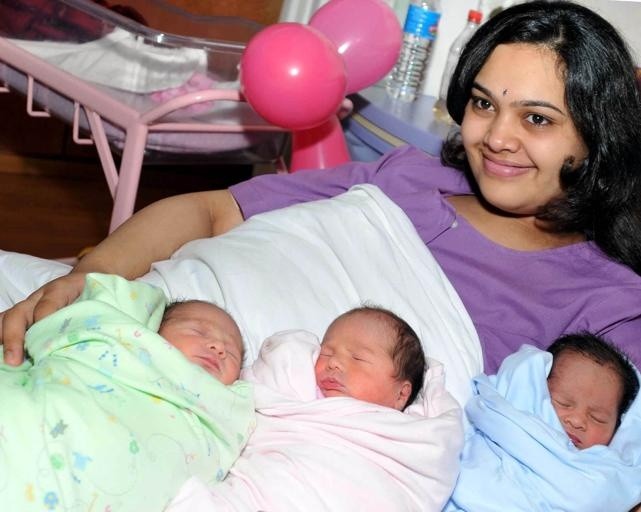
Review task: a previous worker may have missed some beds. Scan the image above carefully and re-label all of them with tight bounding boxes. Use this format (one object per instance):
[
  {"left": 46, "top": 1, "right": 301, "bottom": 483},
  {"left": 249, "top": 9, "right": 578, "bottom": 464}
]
[{"left": 1, "top": 2, "right": 352, "bottom": 238}]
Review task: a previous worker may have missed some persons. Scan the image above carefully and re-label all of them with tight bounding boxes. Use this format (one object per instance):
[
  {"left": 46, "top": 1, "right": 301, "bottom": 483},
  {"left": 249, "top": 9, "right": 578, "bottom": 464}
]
[
  {"left": 545, "top": 330, "right": 640, "bottom": 450},
  {"left": 313, "top": 306, "right": 424, "bottom": 413},
  {"left": 158, "top": 299, "right": 243, "bottom": 387},
  {"left": 0, "top": 0, "right": 641, "bottom": 384}
]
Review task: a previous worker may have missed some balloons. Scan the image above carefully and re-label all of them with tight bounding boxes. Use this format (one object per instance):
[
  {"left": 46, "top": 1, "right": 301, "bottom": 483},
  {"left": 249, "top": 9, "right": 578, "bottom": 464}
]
[
  {"left": 308, "top": 0, "right": 403, "bottom": 96},
  {"left": 239, "top": 22, "right": 347, "bottom": 130},
  {"left": 290, "top": 115, "right": 351, "bottom": 174}
]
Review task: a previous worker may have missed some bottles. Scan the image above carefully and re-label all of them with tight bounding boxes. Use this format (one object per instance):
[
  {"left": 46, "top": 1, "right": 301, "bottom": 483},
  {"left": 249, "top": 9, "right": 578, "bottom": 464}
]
[{"left": 387, "top": 1, "right": 483, "bottom": 125}]
